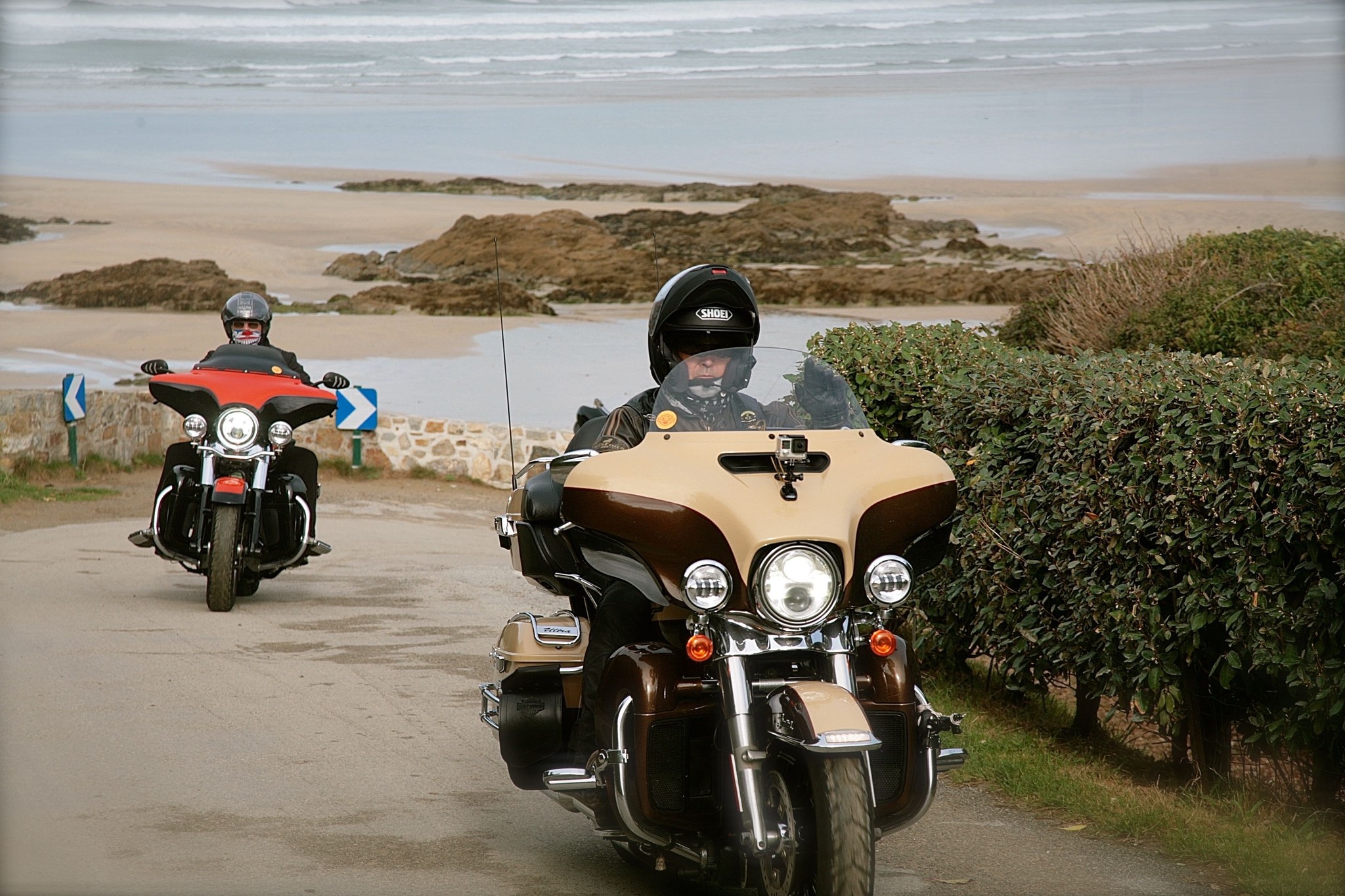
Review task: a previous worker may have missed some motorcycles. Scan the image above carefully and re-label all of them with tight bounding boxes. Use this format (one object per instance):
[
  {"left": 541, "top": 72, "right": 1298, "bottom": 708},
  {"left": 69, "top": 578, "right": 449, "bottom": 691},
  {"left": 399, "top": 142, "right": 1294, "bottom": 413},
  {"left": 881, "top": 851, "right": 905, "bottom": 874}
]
[
  {"left": 128, "top": 344, "right": 351, "bottom": 612},
  {"left": 479, "top": 348, "right": 969, "bottom": 896}
]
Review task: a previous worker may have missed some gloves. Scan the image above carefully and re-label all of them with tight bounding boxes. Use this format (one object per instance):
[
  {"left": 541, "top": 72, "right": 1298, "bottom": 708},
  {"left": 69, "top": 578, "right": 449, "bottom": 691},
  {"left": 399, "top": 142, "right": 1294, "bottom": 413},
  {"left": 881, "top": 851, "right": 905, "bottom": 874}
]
[{"left": 793, "top": 358, "right": 847, "bottom": 425}]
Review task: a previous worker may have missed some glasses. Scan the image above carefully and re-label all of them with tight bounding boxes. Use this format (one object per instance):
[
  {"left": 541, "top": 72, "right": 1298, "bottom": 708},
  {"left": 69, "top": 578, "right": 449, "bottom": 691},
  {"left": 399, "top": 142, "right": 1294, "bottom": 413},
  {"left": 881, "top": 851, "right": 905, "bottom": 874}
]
[
  {"left": 681, "top": 342, "right": 733, "bottom": 358},
  {"left": 232, "top": 319, "right": 259, "bottom": 330}
]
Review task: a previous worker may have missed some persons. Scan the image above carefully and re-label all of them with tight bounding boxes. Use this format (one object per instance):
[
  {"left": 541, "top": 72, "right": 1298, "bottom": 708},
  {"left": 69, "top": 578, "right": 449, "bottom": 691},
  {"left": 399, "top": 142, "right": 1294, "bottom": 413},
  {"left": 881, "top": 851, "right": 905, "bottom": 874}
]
[
  {"left": 579, "top": 264, "right": 856, "bottom": 762},
  {"left": 150, "top": 291, "right": 318, "bottom": 556}
]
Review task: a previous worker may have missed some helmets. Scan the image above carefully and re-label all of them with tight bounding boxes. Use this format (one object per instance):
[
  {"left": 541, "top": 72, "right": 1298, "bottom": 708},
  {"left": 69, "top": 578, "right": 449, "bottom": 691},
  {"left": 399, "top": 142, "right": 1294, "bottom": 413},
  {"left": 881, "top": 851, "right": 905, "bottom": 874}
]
[
  {"left": 220, "top": 291, "right": 273, "bottom": 346},
  {"left": 648, "top": 263, "right": 760, "bottom": 393}
]
